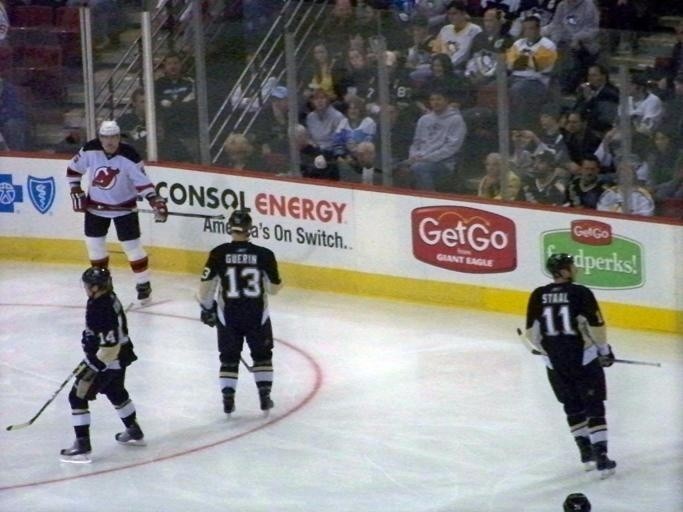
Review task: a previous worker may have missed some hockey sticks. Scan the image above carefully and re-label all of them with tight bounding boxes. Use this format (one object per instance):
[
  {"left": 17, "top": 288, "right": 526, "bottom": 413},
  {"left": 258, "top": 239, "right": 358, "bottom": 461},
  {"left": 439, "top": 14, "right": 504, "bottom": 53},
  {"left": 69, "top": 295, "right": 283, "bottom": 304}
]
[
  {"left": 6, "top": 303, "right": 137, "bottom": 431},
  {"left": 89, "top": 204, "right": 235, "bottom": 220},
  {"left": 515, "top": 328, "right": 662, "bottom": 367},
  {"left": 195, "top": 296, "right": 254, "bottom": 373}
]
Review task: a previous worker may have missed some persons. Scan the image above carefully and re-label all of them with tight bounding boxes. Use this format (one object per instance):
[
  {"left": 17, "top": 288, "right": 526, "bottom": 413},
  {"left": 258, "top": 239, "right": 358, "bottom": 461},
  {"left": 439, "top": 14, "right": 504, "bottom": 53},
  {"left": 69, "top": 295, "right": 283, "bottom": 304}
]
[
  {"left": 1, "top": 2, "right": 682, "bottom": 217},
  {"left": 60, "top": 266, "right": 143, "bottom": 456},
  {"left": 201, "top": 211, "right": 284, "bottom": 411},
  {"left": 68, "top": 120, "right": 169, "bottom": 298},
  {"left": 526, "top": 252, "right": 618, "bottom": 470}
]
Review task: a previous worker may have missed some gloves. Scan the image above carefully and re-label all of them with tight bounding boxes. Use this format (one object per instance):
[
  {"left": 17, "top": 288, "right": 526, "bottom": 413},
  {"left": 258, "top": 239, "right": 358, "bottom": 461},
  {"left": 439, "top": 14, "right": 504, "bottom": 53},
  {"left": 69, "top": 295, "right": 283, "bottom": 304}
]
[
  {"left": 598, "top": 346, "right": 614, "bottom": 366},
  {"left": 200, "top": 301, "right": 217, "bottom": 326},
  {"left": 70, "top": 191, "right": 87, "bottom": 212},
  {"left": 150, "top": 197, "right": 168, "bottom": 222}
]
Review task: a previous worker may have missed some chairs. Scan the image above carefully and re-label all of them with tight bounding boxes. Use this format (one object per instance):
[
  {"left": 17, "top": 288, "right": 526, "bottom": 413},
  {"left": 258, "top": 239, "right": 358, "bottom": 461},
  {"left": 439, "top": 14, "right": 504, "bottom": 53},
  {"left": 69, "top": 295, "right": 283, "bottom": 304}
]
[{"left": 0, "top": 1, "right": 682, "bottom": 224}]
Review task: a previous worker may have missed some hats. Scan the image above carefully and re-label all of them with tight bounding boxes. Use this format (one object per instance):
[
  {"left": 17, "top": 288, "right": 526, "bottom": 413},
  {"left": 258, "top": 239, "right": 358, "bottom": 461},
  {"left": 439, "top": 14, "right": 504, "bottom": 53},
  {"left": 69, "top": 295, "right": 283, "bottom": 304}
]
[
  {"left": 272, "top": 86, "right": 287, "bottom": 98},
  {"left": 530, "top": 150, "right": 554, "bottom": 162}
]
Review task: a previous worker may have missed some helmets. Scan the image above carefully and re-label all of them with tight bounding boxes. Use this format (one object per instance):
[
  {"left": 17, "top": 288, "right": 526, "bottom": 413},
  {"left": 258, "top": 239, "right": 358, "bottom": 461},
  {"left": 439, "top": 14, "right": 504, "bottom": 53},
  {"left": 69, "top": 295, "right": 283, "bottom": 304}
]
[
  {"left": 83, "top": 267, "right": 109, "bottom": 287},
  {"left": 547, "top": 254, "right": 574, "bottom": 279},
  {"left": 229, "top": 211, "right": 252, "bottom": 233},
  {"left": 99, "top": 120, "right": 120, "bottom": 136}
]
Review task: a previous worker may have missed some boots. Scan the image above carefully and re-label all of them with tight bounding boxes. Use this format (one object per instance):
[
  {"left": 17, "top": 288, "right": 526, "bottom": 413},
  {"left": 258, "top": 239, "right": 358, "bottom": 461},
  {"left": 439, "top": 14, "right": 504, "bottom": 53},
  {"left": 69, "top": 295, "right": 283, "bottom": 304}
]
[
  {"left": 222, "top": 387, "right": 235, "bottom": 412},
  {"left": 115, "top": 422, "right": 143, "bottom": 441},
  {"left": 261, "top": 387, "right": 273, "bottom": 409},
  {"left": 136, "top": 282, "right": 152, "bottom": 299},
  {"left": 593, "top": 445, "right": 616, "bottom": 469},
  {"left": 575, "top": 435, "right": 594, "bottom": 462},
  {"left": 61, "top": 435, "right": 91, "bottom": 455}
]
